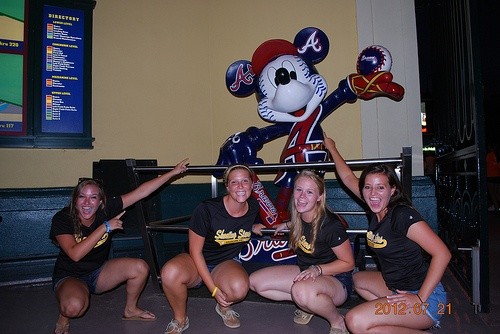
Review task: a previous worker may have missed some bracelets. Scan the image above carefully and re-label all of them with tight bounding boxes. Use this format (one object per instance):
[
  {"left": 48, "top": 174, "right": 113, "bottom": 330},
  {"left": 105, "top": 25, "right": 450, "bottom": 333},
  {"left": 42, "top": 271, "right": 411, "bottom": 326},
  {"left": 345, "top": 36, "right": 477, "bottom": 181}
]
[
  {"left": 316, "top": 265, "right": 322, "bottom": 276},
  {"left": 286, "top": 221, "right": 289, "bottom": 229},
  {"left": 103, "top": 221, "right": 110, "bottom": 232},
  {"left": 416, "top": 293, "right": 424, "bottom": 304},
  {"left": 311, "top": 264, "right": 320, "bottom": 276},
  {"left": 212, "top": 287, "right": 218, "bottom": 296}
]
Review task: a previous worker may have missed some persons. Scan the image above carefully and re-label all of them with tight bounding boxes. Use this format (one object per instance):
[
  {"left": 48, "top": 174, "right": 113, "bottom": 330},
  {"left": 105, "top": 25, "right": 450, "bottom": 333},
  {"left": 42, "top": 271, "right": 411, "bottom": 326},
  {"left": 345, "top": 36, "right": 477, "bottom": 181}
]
[
  {"left": 161, "top": 163, "right": 267, "bottom": 334},
  {"left": 249, "top": 170, "right": 355, "bottom": 334},
  {"left": 485, "top": 142, "right": 500, "bottom": 211},
  {"left": 324, "top": 131, "right": 452, "bottom": 334},
  {"left": 49, "top": 157, "right": 189, "bottom": 334}
]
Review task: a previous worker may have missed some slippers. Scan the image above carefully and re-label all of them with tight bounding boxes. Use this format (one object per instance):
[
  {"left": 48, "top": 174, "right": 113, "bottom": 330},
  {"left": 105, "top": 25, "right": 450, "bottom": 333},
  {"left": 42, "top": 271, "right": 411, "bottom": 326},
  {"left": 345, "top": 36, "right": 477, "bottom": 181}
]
[
  {"left": 55, "top": 313, "right": 69, "bottom": 334},
  {"left": 121, "top": 310, "right": 157, "bottom": 321}
]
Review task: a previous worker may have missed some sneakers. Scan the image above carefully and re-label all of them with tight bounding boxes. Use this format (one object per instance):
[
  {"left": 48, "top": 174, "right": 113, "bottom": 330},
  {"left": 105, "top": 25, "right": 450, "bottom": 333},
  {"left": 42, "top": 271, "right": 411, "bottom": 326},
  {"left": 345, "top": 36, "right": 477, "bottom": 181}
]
[
  {"left": 293, "top": 308, "right": 314, "bottom": 324},
  {"left": 164, "top": 314, "right": 190, "bottom": 334},
  {"left": 329, "top": 314, "right": 349, "bottom": 334},
  {"left": 215, "top": 302, "right": 240, "bottom": 328}
]
